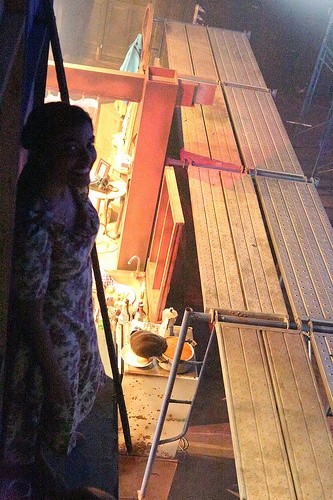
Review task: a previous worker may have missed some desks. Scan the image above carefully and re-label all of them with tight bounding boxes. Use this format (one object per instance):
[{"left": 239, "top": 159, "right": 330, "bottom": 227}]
[
  {"left": 88, "top": 170, "right": 127, "bottom": 213},
  {"left": 92, "top": 269, "right": 149, "bottom": 379}
]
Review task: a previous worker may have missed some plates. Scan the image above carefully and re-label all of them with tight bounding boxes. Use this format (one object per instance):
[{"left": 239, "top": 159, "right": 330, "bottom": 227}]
[
  {"left": 119, "top": 345, "right": 155, "bottom": 367},
  {"left": 103, "top": 282, "right": 135, "bottom": 306}
]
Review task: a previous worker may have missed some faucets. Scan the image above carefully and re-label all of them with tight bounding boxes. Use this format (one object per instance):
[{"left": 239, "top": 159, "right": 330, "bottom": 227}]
[{"left": 127, "top": 255, "right": 141, "bottom": 275}]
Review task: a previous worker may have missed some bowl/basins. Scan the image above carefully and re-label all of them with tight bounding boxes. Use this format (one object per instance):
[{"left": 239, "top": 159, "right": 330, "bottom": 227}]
[{"left": 158, "top": 337, "right": 197, "bottom": 372}]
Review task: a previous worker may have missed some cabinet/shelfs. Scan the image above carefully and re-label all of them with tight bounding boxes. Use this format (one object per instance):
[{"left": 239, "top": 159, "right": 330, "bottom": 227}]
[{"left": 84, "top": 0, "right": 157, "bottom": 65}]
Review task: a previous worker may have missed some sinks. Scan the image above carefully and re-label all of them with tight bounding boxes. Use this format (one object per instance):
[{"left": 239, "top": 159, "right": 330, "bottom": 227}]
[{"left": 104, "top": 269, "right": 148, "bottom": 319}]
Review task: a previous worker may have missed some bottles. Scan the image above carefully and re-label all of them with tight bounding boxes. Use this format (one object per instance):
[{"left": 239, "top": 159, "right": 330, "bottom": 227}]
[
  {"left": 132, "top": 302, "right": 148, "bottom": 320},
  {"left": 117, "top": 305, "right": 129, "bottom": 343}
]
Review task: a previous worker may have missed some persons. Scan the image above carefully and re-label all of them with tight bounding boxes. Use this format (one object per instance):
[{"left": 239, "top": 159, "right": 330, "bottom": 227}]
[{"left": 0, "top": 102, "right": 107, "bottom": 500}]
